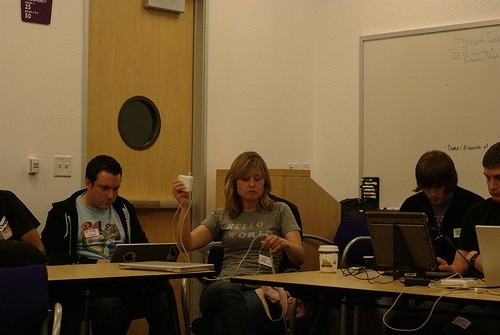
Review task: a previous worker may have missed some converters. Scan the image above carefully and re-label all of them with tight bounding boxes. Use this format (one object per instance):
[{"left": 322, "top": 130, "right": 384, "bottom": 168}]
[{"left": 404, "top": 277, "right": 430, "bottom": 287}]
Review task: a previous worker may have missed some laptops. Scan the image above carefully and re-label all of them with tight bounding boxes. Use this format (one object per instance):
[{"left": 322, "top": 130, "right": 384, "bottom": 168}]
[
  {"left": 109, "top": 243, "right": 180, "bottom": 263},
  {"left": 365, "top": 212, "right": 451, "bottom": 276},
  {"left": 475, "top": 226, "right": 500, "bottom": 286}
]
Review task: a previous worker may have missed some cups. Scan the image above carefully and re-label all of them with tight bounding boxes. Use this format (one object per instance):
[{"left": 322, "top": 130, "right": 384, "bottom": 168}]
[{"left": 318, "top": 245, "right": 339, "bottom": 273}]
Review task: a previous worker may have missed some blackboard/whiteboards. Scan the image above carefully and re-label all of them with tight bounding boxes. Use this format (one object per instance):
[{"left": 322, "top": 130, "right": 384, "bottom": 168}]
[{"left": 358, "top": 19, "right": 500, "bottom": 210}]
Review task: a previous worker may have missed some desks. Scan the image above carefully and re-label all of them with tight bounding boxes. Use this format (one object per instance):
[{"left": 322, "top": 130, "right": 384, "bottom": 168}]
[
  {"left": 46, "top": 260, "right": 215, "bottom": 335},
  {"left": 230, "top": 269, "right": 500, "bottom": 335}
]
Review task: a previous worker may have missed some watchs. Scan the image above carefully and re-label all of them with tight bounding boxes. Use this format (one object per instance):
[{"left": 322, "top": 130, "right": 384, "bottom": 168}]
[{"left": 470, "top": 253, "right": 480, "bottom": 266}]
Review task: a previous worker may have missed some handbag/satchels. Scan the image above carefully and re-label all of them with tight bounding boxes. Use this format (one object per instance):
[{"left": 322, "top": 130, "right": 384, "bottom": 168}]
[{"left": 254, "top": 284, "right": 306, "bottom": 323}]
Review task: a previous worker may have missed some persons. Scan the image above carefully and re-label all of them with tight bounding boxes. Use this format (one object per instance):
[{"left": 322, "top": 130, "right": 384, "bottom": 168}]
[
  {"left": 39, "top": 155, "right": 180, "bottom": 335},
  {"left": 0, "top": 190, "right": 45, "bottom": 254},
  {"left": 438, "top": 143, "right": 500, "bottom": 315},
  {"left": 172, "top": 152, "right": 304, "bottom": 335},
  {"left": 400, "top": 151, "right": 485, "bottom": 266}
]
